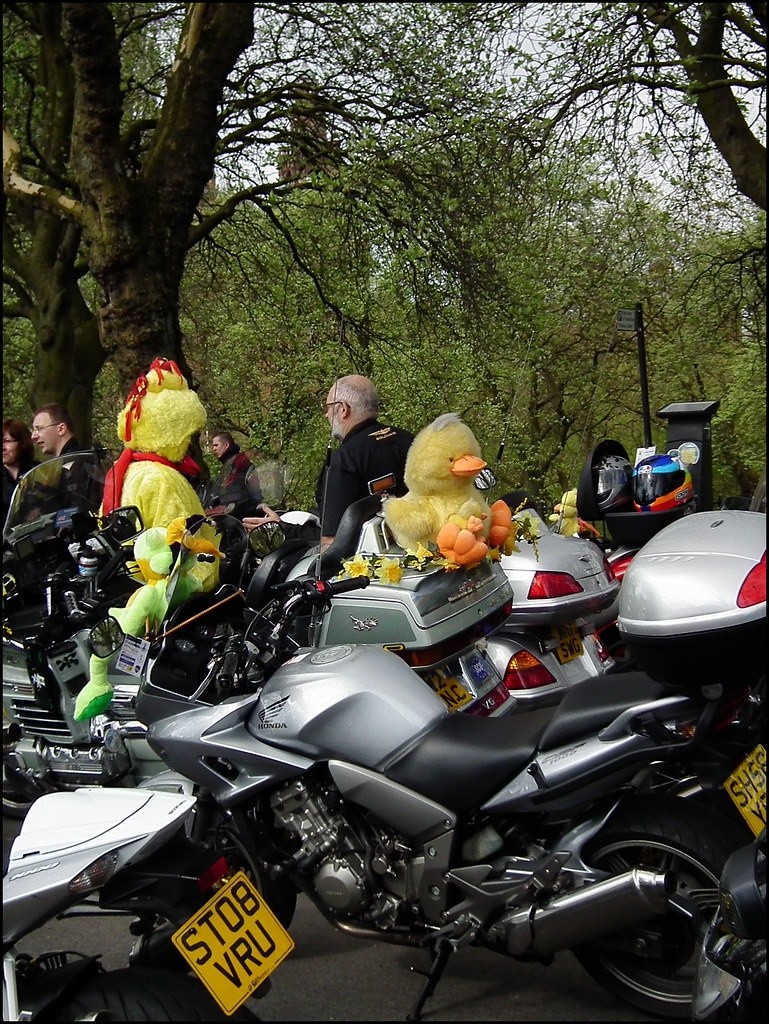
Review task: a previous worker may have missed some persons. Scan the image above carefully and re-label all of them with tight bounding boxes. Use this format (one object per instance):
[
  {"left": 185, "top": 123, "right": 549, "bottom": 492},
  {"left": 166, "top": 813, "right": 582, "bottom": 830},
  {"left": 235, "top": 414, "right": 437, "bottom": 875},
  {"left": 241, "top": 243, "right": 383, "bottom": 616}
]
[
  {"left": 211, "top": 432, "right": 264, "bottom": 522},
  {"left": 314, "top": 374, "right": 416, "bottom": 581},
  {"left": 0, "top": 418, "right": 44, "bottom": 540},
  {"left": 31, "top": 402, "right": 113, "bottom": 530}
]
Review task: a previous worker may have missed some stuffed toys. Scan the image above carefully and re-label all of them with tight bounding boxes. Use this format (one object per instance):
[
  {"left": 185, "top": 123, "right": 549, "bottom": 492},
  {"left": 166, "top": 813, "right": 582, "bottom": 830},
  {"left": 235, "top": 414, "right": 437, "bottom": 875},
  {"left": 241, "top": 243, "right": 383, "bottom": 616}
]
[
  {"left": 549, "top": 486, "right": 600, "bottom": 537},
  {"left": 73, "top": 519, "right": 225, "bottom": 721},
  {"left": 381, "top": 412, "right": 512, "bottom": 566},
  {"left": 98, "top": 356, "right": 220, "bottom": 592}
]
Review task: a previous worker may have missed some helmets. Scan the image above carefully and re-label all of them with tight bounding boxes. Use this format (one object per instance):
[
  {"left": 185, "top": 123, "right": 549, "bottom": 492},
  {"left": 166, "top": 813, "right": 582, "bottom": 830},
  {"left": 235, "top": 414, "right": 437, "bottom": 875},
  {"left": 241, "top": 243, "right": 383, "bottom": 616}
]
[{"left": 591, "top": 453, "right": 693, "bottom": 513}]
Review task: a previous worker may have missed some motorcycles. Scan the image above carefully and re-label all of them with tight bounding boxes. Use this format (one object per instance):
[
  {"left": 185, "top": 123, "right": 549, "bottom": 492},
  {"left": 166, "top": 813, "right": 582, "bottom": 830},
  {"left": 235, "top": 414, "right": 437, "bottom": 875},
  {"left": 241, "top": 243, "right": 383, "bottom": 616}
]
[{"left": 0, "top": 446, "right": 769, "bottom": 1023}]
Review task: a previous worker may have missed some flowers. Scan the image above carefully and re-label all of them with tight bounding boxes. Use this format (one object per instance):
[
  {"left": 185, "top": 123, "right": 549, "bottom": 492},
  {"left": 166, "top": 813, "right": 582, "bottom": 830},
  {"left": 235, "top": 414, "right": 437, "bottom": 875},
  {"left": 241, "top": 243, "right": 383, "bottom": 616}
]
[{"left": 335, "top": 494, "right": 542, "bottom": 586}]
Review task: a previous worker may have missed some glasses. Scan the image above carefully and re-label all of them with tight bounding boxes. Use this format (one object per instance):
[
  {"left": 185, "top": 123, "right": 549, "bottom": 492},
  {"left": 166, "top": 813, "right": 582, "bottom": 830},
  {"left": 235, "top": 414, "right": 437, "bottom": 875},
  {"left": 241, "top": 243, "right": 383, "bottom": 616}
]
[
  {"left": 28, "top": 421, "right": 65, "bottom": 433},
  {"left": 320, "top": 400, "right": 350, "bottom": 413}
]
